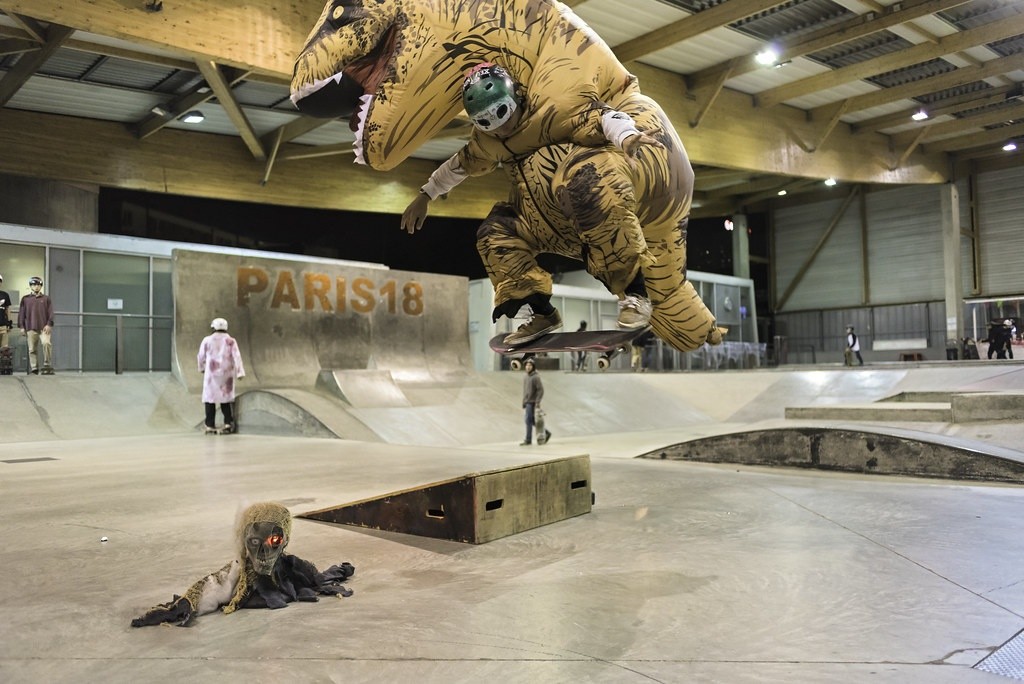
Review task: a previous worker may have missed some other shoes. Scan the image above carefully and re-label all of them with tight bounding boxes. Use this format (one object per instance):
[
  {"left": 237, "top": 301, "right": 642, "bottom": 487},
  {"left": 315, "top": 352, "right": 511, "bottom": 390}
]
[
  {"left": 521, "top": 441, "right": 531, "bottom": 445},
  {"left": 205, "top": 426, "right": 217, "bottom": 433},
  {"left": 219, "top": 425, "right": 231, "bottom": 433},
  {"left": 858, "top": 362, "right": 864, "bottom": 366},
  {"left": 545, "top": 432, "right": 551, "bottom": 441},
  {"left": 43, "top": 365, "right": 51, "bottom": 370},
  {"left": 31, "top": 369, "right": 38, "bottom": 375}
]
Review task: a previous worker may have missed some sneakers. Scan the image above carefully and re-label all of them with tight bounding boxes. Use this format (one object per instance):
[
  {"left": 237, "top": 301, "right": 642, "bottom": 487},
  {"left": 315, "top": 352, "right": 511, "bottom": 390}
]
[
  {"left": 616, "top": 296, "right": 653, "bottom": 329},
  {"left": 503, "top": 307, "right": 564, "bottom": 345}
]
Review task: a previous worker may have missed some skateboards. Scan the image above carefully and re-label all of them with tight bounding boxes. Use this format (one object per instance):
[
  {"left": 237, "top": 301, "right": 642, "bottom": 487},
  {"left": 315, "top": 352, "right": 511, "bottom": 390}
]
[
  {"left": 488, "top": 324, "right": 654, "bottom": 370},
  {"left": 200, "top": 423, "right": 236, "bottom": 435},
  {"left": 0, "top": 345, "right": 14, "bottom": 375},
  {"left": 845, "top": 346, "right": 853, "bottom": 367},
  {"left": 41, "top": 366, "right": 56, "bottom": 375},
  {"left": 534, "top": 406, "right": 547, "bottom": 447}
]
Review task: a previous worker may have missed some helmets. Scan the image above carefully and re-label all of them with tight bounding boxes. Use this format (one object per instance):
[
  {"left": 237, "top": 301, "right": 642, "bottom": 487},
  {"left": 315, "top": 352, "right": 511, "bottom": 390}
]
[
  {"left": 462, "top": 62, "right": 522, "bottom": 131},
  {"left": 525, "top": 359, "right": 535, "bottom": 369},
  {"left": 846, "top": 324, "right": 855, "bottom": 329},
  {"left": 210, "top": 317, "right": 228, "bottom": 331},
  {"left": 30, "top": 277, "right": 42, "bottom": 285}
]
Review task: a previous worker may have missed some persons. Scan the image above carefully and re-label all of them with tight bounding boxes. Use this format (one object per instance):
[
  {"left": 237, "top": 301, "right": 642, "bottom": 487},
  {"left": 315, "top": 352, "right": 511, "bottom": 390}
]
[
  {"left": 244, "top": 503, "right": 293, "bottom": 576},
  {"left": 197, "top": 317, "right": 246, "bottom": 431},
  {"left": 999, "top": 318, "right": 1017, "bottom": 359},
  {"left": 17, "top": 277, "right": 57, "bottom": 375},
  {"left": 980, "top": 321, "right": 1003, "bottom": 359},
  {"left": 574, "top": 320, "right": 587, "bottom": 373},
  {"left": 630, "top": 346, "right": 654, "bottom": 372},
  {"left": 0, "top": 275, "right": 13, "bottom": 375},
  {"left": 401, "top": 62, "right": 666, "bottom": 345},
  {"left": 519, "top": 359, "right": 551, "bottom": 446},
  {"left": 842, "top": 324, "right": 864, "bottom": 366}
]
